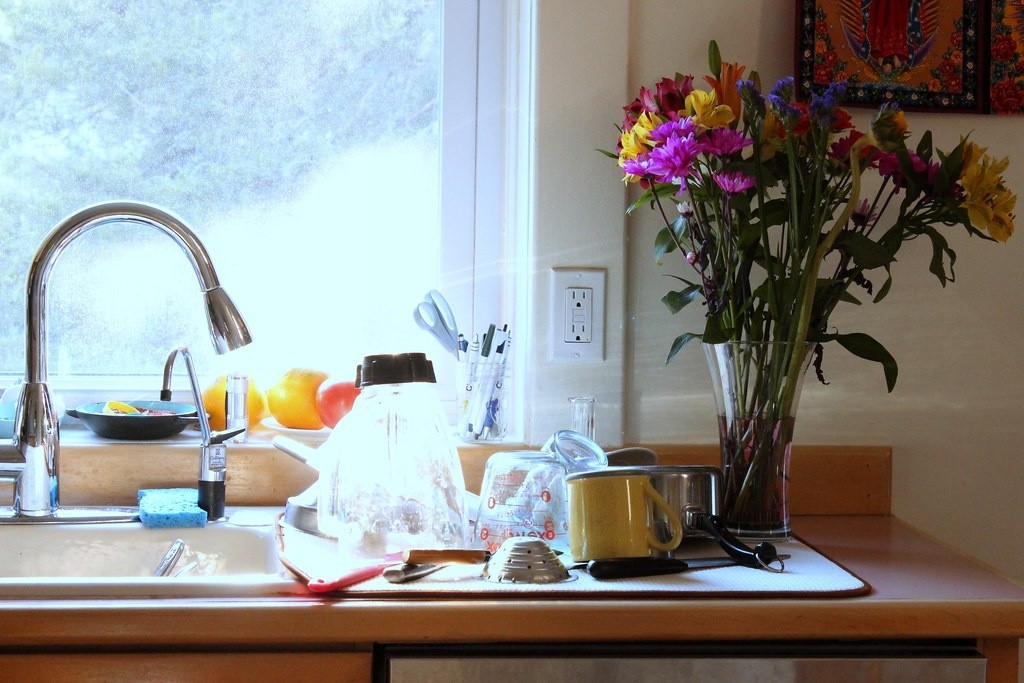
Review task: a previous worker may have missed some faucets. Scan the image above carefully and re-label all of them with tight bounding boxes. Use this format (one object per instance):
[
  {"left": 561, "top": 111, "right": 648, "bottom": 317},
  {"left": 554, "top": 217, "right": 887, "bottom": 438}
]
[
  {"left": 0, "top": 200, "right": 252, "bottom": 515},
  {"left": 158, "top": 346, "right": 244, "bottom": 481}
]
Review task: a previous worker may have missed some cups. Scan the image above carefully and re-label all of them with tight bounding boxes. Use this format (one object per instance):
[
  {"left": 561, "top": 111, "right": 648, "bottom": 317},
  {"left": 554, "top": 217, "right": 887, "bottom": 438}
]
[
  {"left": 472, "top": 450, "right": 571, "bottom": 571},
  {"left": 514, "top": 429, "right": 609, "bottom": 541},
  {"left": 457, "top": 362, "right": 512, "bottom": 444},
  {"left": 566, "top": 468, "right": 683, "bottom": 559}
]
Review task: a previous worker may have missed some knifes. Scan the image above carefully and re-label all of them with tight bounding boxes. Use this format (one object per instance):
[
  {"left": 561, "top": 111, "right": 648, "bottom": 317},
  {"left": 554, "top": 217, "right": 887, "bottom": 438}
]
[
  {"left": 587, "top": 555, "right": 792, "bottom": 578},
  {"left": 402, "top": 549, "right": 563, "bottom": 563}
]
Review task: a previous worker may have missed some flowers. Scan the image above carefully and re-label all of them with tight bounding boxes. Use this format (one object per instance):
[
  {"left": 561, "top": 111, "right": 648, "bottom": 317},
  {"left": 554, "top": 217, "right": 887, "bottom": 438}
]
[{"left": 591, "top": 37, "right": 1019, "bottom": 395}]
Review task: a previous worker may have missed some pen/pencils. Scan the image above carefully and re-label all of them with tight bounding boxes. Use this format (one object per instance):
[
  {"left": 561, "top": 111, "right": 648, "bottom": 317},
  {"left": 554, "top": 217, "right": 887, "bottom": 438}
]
[{"left": 457, "top": 323, "right": 513, "bottom": 442}]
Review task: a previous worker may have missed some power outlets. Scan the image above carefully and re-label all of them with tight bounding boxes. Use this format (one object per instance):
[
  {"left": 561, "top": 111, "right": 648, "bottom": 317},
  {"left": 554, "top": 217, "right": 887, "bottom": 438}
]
[{"left": 545, "top": 266, "right": 609, "bottom": 364}]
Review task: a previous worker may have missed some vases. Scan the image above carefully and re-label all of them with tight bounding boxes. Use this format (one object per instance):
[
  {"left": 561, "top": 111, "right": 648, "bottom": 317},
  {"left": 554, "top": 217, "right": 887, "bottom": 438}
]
[{"left": 697, "top": 339, "right": 817, "bottom": 545}]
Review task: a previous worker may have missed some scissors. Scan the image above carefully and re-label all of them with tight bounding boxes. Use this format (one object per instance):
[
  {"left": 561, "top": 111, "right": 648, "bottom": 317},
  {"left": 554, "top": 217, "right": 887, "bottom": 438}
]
[{"left": 411, "top": 288, "right": 460, "bottom": 363}]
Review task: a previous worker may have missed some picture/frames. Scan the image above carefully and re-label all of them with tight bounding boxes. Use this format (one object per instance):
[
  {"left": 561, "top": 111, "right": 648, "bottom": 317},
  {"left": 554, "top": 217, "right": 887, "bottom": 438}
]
[
  {"left": 797, "top": 0, "right": 979, "bottom": 111},
  {"left": 982, "top": 0, "right": 1024, "bottom": 116}
]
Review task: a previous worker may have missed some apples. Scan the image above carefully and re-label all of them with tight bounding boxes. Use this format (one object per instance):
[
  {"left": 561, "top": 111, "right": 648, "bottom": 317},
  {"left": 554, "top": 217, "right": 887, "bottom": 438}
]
[{"left": 200, "top": 365, "right": 365, "bottom": 432}]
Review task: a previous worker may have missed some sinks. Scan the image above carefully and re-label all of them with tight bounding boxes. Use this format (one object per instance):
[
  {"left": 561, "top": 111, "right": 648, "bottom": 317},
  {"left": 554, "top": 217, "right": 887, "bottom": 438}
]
[{"left": 0, "top": 520, "right": 295, "bottom": 600}]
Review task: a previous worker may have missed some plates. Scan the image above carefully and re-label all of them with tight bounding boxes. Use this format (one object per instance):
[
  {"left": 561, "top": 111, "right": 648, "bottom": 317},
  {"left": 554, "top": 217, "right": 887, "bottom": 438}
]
[
  {"left": 260, "top": 417, "right": 333, "bottom": 443},
  {"left": 70, "top": 398, "right": 208, "bottom": 439}
]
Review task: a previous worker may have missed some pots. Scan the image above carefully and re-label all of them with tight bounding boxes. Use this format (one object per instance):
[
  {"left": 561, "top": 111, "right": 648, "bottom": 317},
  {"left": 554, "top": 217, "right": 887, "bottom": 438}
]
[{"left": 634, "top": 462, "right": 786, "bottom": 573}]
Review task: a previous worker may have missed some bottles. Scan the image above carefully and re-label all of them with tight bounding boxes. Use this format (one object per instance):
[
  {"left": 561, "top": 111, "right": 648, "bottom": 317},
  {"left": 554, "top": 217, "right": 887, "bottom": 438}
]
[{"left": 571, "top": 398, "right": 594, "bottom": 442}]
